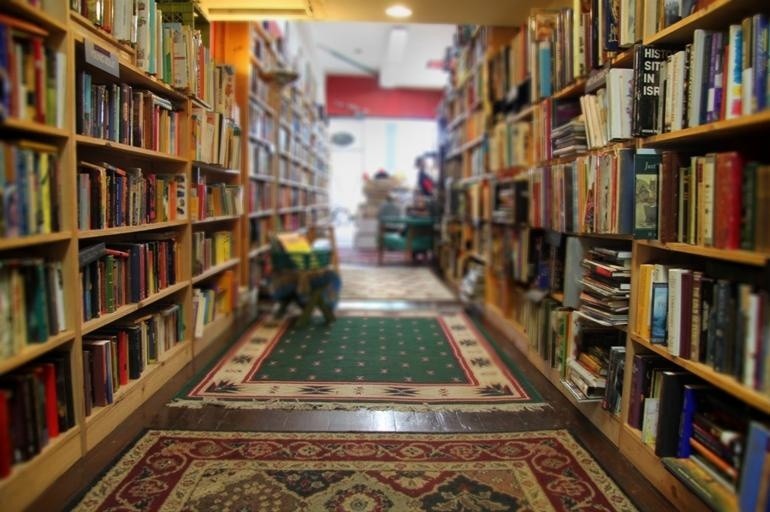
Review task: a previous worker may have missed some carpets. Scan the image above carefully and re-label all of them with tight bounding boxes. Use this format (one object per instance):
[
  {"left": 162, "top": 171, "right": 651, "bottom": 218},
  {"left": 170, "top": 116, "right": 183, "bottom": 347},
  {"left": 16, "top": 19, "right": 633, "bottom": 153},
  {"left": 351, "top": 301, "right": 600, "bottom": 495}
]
[
  {"left": 62, "top": 427, "right": 639, "bottom": 512},
  {"left": 339, "top": 265, "right": 458, "bottom": 302},
  {"left": 165, "top": 305, "right": 553, "bottom": 412}
]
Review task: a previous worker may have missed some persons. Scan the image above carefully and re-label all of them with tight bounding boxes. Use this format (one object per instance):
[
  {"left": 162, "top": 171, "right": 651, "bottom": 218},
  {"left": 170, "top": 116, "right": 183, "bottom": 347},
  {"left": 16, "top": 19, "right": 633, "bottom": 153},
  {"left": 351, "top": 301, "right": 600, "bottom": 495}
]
[{"left": 413, "top": 156, "right": 433, "bottom": 206}]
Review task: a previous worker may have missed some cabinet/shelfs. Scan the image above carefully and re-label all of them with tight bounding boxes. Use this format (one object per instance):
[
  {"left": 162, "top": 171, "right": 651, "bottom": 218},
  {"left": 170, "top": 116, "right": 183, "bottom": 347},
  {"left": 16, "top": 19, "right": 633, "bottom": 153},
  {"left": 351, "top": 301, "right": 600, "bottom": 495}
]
[
  {"left": 245, "top": 21, "right": 332, "bottom": 332},
  {"left": 437, "top": 23, "right": 529, "bottom": 360},
  {"left": 0, "top": 0, "right": 82, "bottom": 512},
  {"left": 190, "top": 0, "right": 246, "bottom": 378},
  {"left": 82, "top": 0, "right": 190, "bottom": 478},
  {"left": 635, "top": 0, "right": 770, "bottom": 511},
  {"left": 529, "top": 0, "right": 635, "bottom": 457}
]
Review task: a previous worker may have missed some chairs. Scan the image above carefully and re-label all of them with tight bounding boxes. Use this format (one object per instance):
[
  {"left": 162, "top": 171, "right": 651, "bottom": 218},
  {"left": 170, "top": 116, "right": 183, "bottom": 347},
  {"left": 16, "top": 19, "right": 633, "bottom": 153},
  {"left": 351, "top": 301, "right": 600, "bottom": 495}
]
[{"left": 378, "top": 207, "right": 435, "bottom": 265}]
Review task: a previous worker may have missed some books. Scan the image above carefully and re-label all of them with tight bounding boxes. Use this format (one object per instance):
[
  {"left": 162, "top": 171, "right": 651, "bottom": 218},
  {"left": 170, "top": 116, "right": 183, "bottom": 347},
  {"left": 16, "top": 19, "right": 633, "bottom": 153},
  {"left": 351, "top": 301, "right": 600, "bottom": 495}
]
[
  {"left": 438, "top": 1, "right": 770, "bottom": 506},
  {"left": 530, "top": 2, "right": 767, "bottom": 512},
  {"left": 1, "top": 0, "right": 240, "bottom": 479},
  {"left": 248, "top": 22, "right": 330, "bottom": 300},
  {"left": 357, "top": 165, "right": 438, "bottom": 270},
  {"left": 439, "top": 22, "right": 534, "bottom": 339}
]
